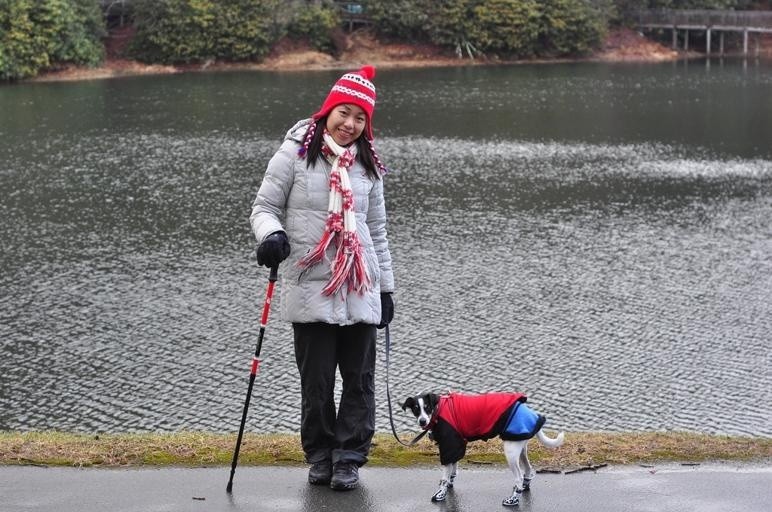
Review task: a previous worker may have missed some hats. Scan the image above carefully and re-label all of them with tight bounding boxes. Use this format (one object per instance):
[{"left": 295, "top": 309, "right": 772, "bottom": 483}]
[{"left": 312, "top": 66, "right": 377, "bottom": 141}]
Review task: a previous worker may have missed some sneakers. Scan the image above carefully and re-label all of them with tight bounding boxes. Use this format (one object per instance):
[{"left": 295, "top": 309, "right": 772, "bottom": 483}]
[
  {"left": 330, "top": 463, "right": 359, "bottom": 489},
  {"left": 309, "top": 461, "right": 333, "bottom": 483}
]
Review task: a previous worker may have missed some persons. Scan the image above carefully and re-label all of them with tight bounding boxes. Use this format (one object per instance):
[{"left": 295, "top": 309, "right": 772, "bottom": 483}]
[{"left": 248, "top": 65, "right": 397, "bottom": 495}]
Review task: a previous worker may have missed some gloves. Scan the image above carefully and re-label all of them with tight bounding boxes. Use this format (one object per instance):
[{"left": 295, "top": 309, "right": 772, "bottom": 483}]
[
  {"left": 257, "top": 234, "right": 290, "bottom": 268},
  {"left": 376, "top": 295, "right": 394, "bottom": 329}
]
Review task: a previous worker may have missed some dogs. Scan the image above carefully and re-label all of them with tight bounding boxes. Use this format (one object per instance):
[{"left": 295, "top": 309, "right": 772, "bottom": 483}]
[{"left": 396, "top": 391, "right": 565, "bottom": 506}]
